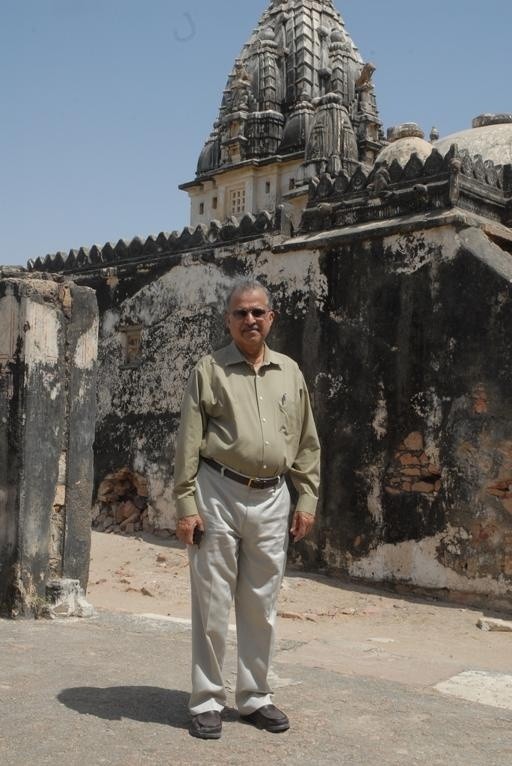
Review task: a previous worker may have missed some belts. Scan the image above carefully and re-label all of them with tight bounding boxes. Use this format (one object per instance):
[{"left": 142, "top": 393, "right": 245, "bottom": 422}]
[{"left": 199, "top": 455, "right": 285, "bottom": 489}]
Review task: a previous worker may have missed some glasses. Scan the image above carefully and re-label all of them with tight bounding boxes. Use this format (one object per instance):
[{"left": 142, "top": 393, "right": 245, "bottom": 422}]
[{"left": 231, "top": 309, "right": 265, "bottom": 318}]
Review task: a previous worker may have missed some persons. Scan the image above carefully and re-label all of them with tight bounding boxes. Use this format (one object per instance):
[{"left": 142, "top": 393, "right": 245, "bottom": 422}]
[{"left": 170, "top": 278, "right": 323, "bottom": 738}]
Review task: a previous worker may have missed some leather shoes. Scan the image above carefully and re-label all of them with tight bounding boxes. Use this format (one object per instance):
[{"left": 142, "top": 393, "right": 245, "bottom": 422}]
[
  {"left": 189, "top": 710, "right": 222, "bottom": 738},
  {"left": 236, "top": 704, "right": 290, "bottom": 732}
]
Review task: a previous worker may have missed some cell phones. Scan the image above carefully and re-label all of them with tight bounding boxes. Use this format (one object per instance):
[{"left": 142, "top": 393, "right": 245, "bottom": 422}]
[{"left": 193, "top": 528, "right": 203, "bottom": 545}]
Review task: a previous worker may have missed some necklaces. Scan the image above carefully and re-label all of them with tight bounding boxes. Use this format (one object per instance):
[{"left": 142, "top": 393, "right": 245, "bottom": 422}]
[{"left": 242, "top": 351, "right": 263, "bottom": 364}]
